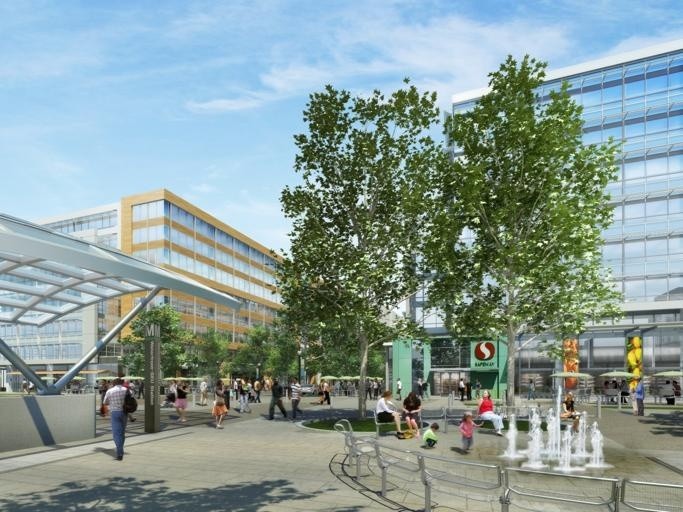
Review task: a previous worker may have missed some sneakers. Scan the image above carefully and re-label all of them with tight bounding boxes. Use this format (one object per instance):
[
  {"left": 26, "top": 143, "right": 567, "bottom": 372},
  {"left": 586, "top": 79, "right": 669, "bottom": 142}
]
[
  {"left": 215, "top": 425, "right": 224, "bottom": 430},
  {"left": 176, "top": 417, "right": 187, "bottom": 423}
]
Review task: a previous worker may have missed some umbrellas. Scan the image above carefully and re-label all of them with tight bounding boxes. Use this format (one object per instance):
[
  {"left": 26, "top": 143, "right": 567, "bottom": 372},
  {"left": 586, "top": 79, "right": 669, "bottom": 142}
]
[
  {"left": 71, "top": 376, "right": 87, "bottom": 380},
  {"left": 652, "top": 370, "right": 683, "bottom": 380},
  {"left": 600, "top": 370, "right": 634, "bottom": 380},
  {"left": 548, "top": 372, "right": 595, "bottom": 388},
  {"left": 40, "top": 376, "right": 57, "bottom": 381}
]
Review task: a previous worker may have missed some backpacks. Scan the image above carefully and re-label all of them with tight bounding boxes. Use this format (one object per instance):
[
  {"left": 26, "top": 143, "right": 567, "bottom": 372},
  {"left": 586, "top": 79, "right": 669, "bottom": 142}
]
[{"left": 122, "top": 388, "right": 138, "bottom": 413}]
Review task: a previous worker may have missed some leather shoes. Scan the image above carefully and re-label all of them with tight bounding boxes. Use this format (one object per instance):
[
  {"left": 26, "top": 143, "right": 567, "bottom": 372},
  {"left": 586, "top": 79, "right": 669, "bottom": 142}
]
[{"left": 116, "top": 456, "right": 123, "bottom": 460}]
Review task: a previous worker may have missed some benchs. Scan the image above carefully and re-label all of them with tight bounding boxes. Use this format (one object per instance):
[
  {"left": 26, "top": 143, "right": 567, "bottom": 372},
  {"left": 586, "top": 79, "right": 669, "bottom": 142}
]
[
  {"left": 373, "top": 405, "right": 586, "bottom": 440},
  {"left": 331, "top": 419, "right": 681, "bottom": 512}
]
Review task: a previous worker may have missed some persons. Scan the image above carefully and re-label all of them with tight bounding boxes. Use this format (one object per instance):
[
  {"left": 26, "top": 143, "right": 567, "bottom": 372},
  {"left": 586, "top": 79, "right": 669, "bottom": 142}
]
[
  {"left": 661, "top": 380, "right": 681, "bottom": 405},
  {"left": 604, "top": 378, "right": 645, "bottom": 416},
  {"left": 459, "top": 411, "right": 484, "bottom": 455},
  {"left": 396, "top": 377, "right": 403, "bottom": 401},
  {"left": 375, "top": 390, "right": 402, "bottom": 433},
  {"left": 561, "top": 392, "right": 579, "bottom": 433},
  {"left": 528, "top": 379, "right": 536, "bottom": 400},
  {"left": 422, "top": 423, "right": 440, "bottom": 448},
  {"left": 417, "top": 377, "right": 425, "bottom": 401},
  {"left": 400, "top": 391, "right": 422, "bottom": 438},
  {"left": 91, "top": 376, "right": 383, "bottom": 429},
  {"left": 22, "top": 378, "right": 35, "bottom": 394},
  {"left": 103, "top": 379, "right": 132, "bottom": 460},
  {"left": 458, "top": 377, "right": 482, "bottom": 401},
  {"left": 477, "top": 389, "right": 504, "bottom": 436}
]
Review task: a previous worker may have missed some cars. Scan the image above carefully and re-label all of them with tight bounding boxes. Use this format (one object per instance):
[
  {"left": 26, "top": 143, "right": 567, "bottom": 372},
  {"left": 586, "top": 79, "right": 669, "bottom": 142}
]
[{"left": 301, "top": 384, "right": 319, "bottom": 396}]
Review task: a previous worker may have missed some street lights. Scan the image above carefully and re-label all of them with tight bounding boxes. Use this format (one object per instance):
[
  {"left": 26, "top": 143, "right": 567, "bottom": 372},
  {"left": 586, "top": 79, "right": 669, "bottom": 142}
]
[{"left": 296, "top": 350, "right": 302, "bottom": 383}]
